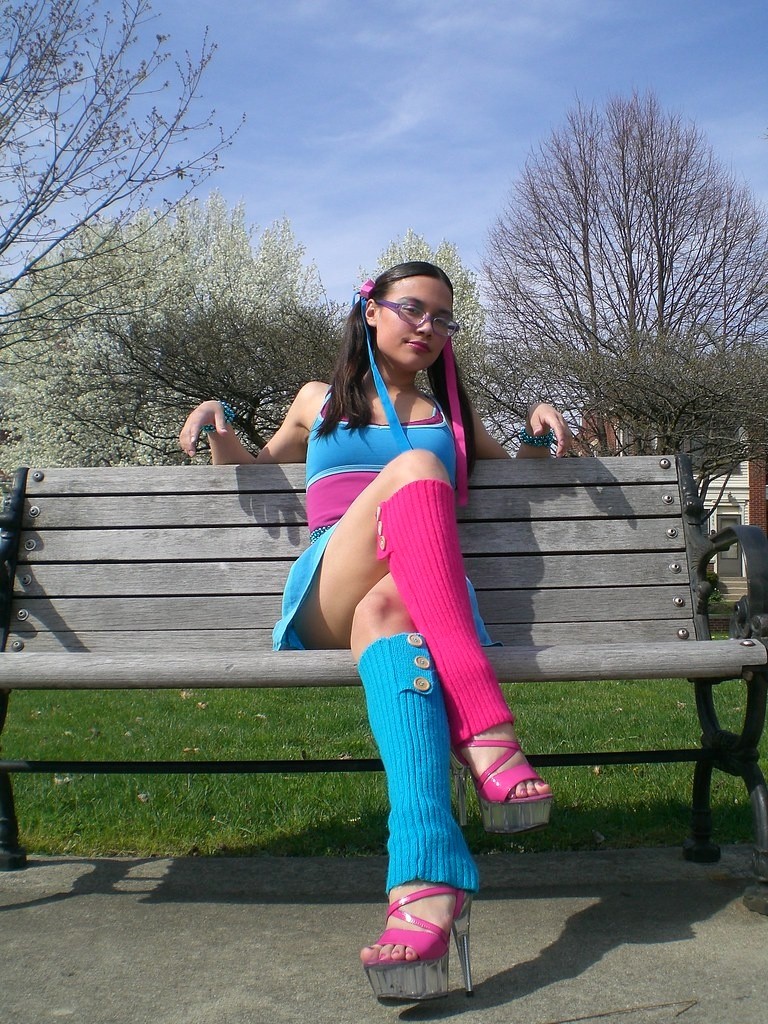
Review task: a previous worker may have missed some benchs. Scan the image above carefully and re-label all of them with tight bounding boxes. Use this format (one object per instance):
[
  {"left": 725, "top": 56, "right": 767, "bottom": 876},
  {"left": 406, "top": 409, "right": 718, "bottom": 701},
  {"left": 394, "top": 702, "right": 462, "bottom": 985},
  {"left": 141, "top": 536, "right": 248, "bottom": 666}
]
[{"left": 0, "top": 451, "right": 768, "bottom": 871}]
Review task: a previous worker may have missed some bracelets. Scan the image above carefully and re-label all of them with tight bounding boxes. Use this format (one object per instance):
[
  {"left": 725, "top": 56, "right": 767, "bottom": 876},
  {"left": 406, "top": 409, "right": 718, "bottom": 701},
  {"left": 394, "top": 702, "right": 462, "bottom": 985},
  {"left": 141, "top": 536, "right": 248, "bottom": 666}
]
[
  {"left": 203, "top": 401, "right": 235, "bottom": 433},
  {"left": 519, "top": 427, "right": 553, "bottom": 448}
]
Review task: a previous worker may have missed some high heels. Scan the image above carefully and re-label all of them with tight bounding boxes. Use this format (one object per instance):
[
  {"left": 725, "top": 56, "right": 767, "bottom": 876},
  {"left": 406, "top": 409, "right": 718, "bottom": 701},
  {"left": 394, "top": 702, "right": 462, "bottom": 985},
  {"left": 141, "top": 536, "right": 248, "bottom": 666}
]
[
  {"left": 364, "top": 885, "right": 475, "bottom": 999},
  {"left": 450, "top": 739, "right": 552, "bottom": 833}
]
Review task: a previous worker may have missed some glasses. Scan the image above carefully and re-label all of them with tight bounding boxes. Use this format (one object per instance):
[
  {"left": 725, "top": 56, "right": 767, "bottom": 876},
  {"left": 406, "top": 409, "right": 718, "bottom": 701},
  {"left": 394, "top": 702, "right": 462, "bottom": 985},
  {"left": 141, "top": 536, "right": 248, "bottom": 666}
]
[{"left": 371, "top": 295, "right": 460, "bottom": 338}]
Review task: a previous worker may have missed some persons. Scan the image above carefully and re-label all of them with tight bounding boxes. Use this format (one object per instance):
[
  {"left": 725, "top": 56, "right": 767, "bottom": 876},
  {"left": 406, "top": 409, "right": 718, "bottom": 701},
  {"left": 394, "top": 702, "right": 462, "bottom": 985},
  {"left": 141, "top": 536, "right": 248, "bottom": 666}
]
[{"left": 180, "top": 261, "right": 573, "bottom": 1007}]
